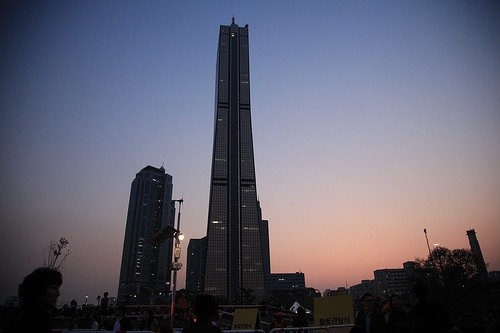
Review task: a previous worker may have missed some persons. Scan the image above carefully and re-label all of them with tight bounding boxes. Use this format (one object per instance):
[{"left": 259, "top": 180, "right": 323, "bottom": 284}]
[
  {"left": 0, "top": 266, "right": 63, "bottom": 333},
  {"left": 89, "top": 317, "right": 99, "bottom": 329},
  {"left": 181, "top": 295, "right": 224, "bottom": 333},
  {"left": 137, "top": 309, "right": 161, "bottom": 333},
  {"left": 347, "top": 294, "right": 455, "bottom": 333},
  {"left": 269, "top": 312, "right": 289, "bottom": 333},
  {"left": 112, "top": 305, "right": 133, "bottom": 330},
  {"left": 292, "top": 307, "right": 308, "bottom": 333}
]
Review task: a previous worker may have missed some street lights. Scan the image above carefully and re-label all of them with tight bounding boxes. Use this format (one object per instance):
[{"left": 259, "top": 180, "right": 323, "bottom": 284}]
[{"left": 169, "top": 199, "right": 184, "bottom": 333}]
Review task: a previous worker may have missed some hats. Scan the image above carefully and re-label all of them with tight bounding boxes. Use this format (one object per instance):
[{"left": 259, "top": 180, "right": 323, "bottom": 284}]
[{"left": 18, "top": 267, "right": 64, "bottom": 305}]
